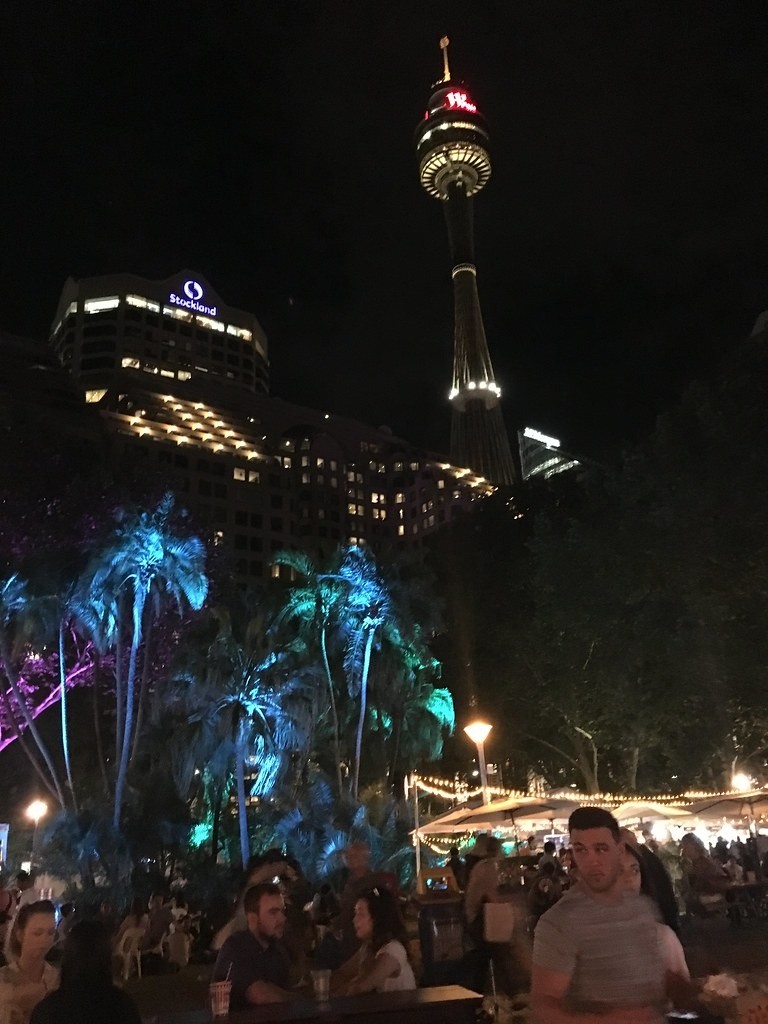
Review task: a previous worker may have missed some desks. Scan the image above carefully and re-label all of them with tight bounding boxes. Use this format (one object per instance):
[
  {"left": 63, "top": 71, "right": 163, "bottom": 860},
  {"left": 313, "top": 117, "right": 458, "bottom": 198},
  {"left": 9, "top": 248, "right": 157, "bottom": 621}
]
[
  {"left": 724, "top": 879, "right": 768, "bottom": 929},
  {"left": 411, "top": 891, "right": 463, "bottom": 986},
  {"left": 157, "top": 984, "right": 485, "bottom": 1024}
]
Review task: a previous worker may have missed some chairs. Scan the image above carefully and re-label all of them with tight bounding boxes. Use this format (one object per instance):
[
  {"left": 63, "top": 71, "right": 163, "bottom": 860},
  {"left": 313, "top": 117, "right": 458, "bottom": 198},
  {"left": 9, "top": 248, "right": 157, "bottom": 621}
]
[
  {"left": 112, "top": 926, "right": 145, "bottom": 981},
  {"left": 142, "top": 922, "right": 176, "bottom": 960}
]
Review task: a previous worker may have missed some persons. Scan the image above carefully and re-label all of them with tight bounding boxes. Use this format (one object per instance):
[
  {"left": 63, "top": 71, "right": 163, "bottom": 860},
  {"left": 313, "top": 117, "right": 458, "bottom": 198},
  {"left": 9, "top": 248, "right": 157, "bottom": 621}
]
[{"left": 0, "top": 808, "right": 768, "bottom": 1024}]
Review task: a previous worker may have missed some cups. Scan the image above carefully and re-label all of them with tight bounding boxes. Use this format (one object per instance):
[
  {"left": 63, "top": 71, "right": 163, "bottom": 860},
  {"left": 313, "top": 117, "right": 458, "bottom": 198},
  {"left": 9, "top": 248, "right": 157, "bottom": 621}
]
[
  {"left": 210, "top": 981, "right": 230, "bottom": 1015},
  {"left": 311, "top": 970, "right": 332, "bottom": 1002}
]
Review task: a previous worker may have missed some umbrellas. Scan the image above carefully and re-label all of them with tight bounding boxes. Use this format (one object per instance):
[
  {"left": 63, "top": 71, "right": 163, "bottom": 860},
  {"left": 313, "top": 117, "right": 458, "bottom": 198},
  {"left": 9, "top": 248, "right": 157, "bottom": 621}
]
[{"left": 409, "top": 784, "right": 768, "bottom": 869}]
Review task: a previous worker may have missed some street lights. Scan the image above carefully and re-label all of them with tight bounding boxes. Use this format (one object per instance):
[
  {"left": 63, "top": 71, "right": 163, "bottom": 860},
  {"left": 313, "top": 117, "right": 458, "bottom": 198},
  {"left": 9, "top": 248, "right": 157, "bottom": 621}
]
[
  {"left": 461, "top": 716, "right": 494, "bottom": 805},
  {"left": 24, "top": 798, "right": 49, "bottom": 874}
]
